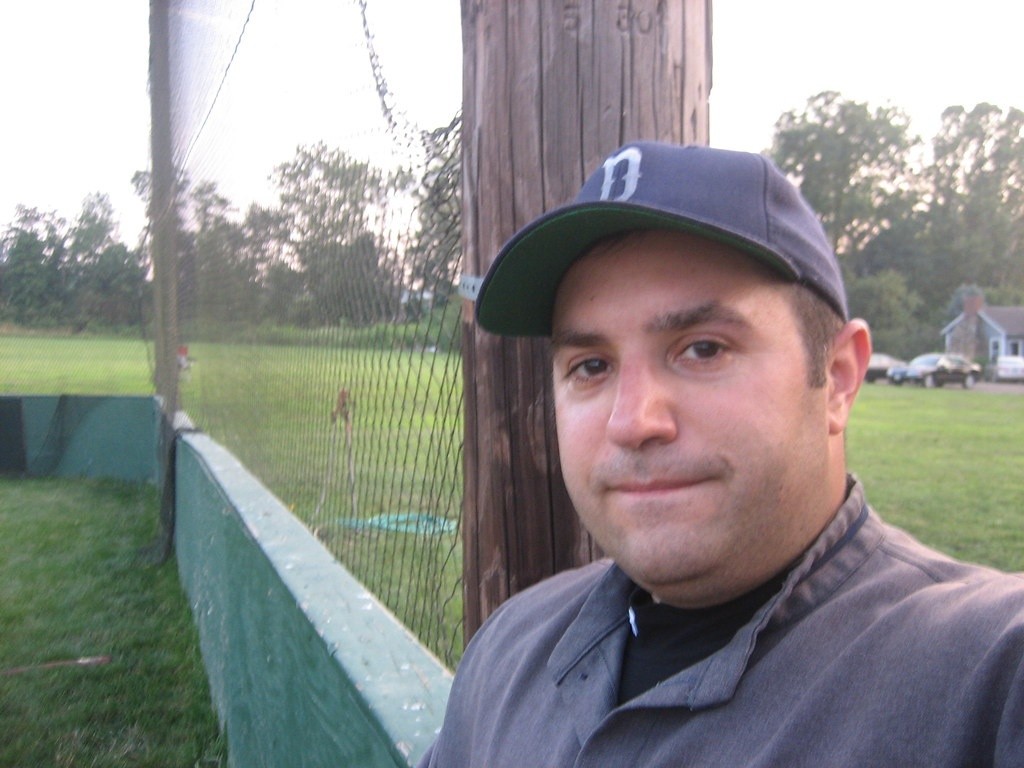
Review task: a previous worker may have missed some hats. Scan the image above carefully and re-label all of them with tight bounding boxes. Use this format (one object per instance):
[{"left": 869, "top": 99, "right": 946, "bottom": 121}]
[{"left": 476, "top": 141, "right": 849, "bottom": 335}]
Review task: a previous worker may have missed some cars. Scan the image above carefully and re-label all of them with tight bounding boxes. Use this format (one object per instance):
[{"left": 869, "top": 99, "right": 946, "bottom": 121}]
[
  {"left": 885, "top": 353, "right": 982, "bottom": 391},
  {"left": 864, "top": 352, "right": 907, "bottom": 385}
]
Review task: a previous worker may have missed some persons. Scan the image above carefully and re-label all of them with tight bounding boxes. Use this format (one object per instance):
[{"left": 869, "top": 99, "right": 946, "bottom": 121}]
[{"left": 422, "top": 142, "right": 1024, "bottom": 768}]
